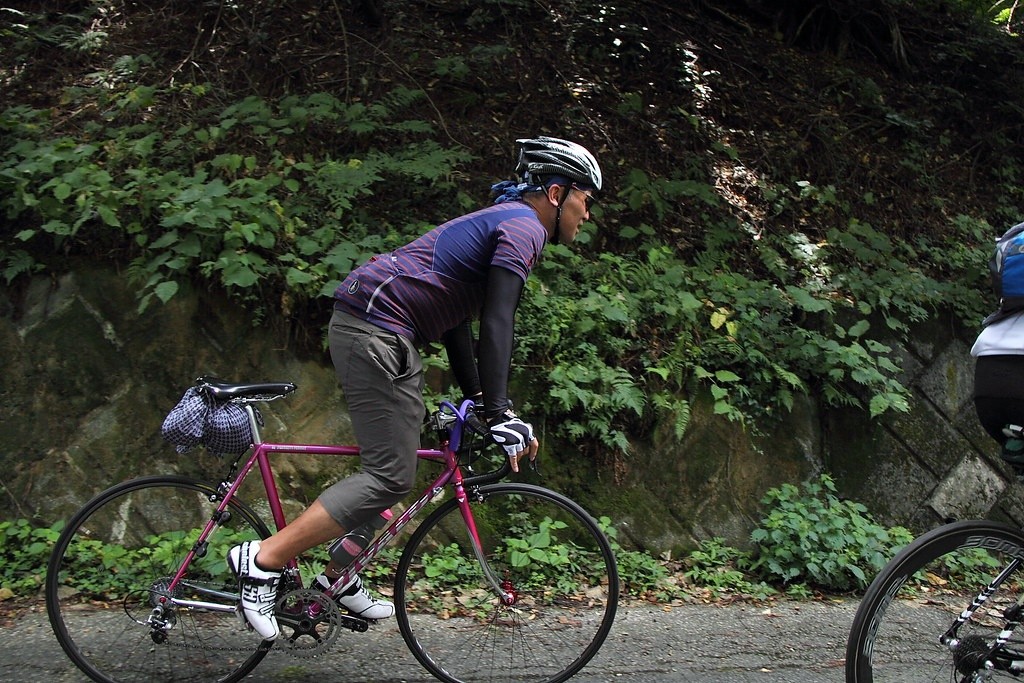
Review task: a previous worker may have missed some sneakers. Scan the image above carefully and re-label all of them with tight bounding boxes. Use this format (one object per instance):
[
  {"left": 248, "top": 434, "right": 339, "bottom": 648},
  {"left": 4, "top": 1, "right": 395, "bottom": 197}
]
[
  {"left": 227, "top": 539, "right": 280, "bottom": 642},
  {"left": 308, "top": 571, "right": 397, "bottom": 621}
]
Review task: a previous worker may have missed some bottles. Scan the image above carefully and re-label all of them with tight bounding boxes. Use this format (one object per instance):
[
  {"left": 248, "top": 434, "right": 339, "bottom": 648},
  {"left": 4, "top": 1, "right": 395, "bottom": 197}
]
[{"left": 327, "top": 507, "right": 393, "bottom": 567}]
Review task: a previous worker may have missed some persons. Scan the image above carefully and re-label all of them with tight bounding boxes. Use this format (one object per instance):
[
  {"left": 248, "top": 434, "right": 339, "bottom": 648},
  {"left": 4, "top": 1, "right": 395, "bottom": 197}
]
[
  {"left": 973, "top": 224, "right": 1023, "bottom": 465},
  {"left": 226, "top": 136, "right": 605, "bottom": 642}
]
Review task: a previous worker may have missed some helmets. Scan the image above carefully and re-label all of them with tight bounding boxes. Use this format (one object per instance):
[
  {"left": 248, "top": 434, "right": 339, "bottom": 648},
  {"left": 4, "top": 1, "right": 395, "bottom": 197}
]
[{"left": 513, "top": 134, "right": 605, "bottom": 191}]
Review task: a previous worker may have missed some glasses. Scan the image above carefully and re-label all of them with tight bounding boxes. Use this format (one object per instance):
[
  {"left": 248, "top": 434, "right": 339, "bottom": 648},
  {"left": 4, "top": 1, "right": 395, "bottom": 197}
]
[{"left": 569, "top": 184, "right": 599, "bottom": 213}]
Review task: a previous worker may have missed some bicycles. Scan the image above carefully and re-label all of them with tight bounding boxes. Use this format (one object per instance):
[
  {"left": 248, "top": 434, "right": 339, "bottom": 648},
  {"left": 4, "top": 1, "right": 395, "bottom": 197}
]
[
  {"left": 844, "top": 420, "right": 1024, "bottom": 683},
  {"left": 43, "top": 375, "right": 618, "bottom": 683}
]
